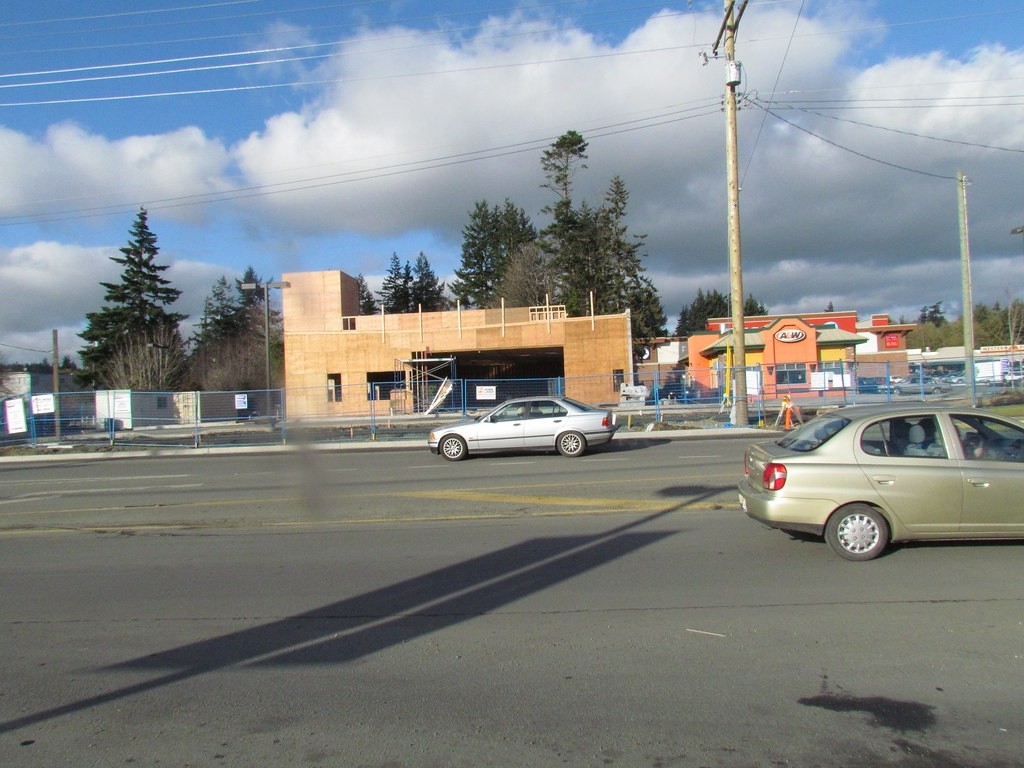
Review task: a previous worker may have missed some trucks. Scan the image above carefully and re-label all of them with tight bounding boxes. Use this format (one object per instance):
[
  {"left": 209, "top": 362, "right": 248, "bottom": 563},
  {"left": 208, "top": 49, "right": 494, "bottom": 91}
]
[{"left": 974, "top": 360, "right": 1024, "bottom": 386}]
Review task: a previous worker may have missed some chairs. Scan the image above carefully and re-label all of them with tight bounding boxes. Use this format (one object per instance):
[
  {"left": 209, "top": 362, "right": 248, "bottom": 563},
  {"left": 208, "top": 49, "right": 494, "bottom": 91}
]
[
  {"left": 903, "top": 425, "right": 931, "bottom": 456},
  {"left": 895, "top": 421, "right": 912, "bottom": 453}
]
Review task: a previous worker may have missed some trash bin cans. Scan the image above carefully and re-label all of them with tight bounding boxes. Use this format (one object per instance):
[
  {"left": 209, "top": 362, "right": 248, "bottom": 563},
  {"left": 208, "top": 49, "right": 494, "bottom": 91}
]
[{"left": 105, "top": 418, "right": 113, "bottom": 431}]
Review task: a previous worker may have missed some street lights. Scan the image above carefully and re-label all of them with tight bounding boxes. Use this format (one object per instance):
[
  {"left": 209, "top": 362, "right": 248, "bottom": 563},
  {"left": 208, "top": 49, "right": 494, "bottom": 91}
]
[{"left": 240, "top": 282, "right": 292, "bottom": 428}]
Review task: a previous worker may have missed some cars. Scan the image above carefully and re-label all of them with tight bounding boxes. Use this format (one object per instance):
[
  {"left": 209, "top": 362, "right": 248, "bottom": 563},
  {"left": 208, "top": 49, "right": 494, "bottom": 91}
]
[
  {"left": 915, "top": 370, "right": 966, "bottom": 383},
  {"left": 737, "top": 401, "right": 1024, "bottom": 561},
  {"left": 428, "top": 396, "right": 616, "bottom": 462},
  {"left": 858, "top": 376, "right": 902, "bottom": 395},
  {"left": 663, "top": 382, "right": 692, "bottom": 398},
  {"left": 891, "top": 375, "right": 951, "bottom": 395}
]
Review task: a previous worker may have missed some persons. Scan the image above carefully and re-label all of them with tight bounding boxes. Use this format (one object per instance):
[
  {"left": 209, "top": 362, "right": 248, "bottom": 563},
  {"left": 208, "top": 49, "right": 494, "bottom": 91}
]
[
  {"left": 517, "top": 406, "right": 525, "bottom": 415},
  {"left": 919, "top": 418, "right": 937, "bottom": 448},
  {"left": 967, "top": 433, "right": 984, "bottom": 458}
]
